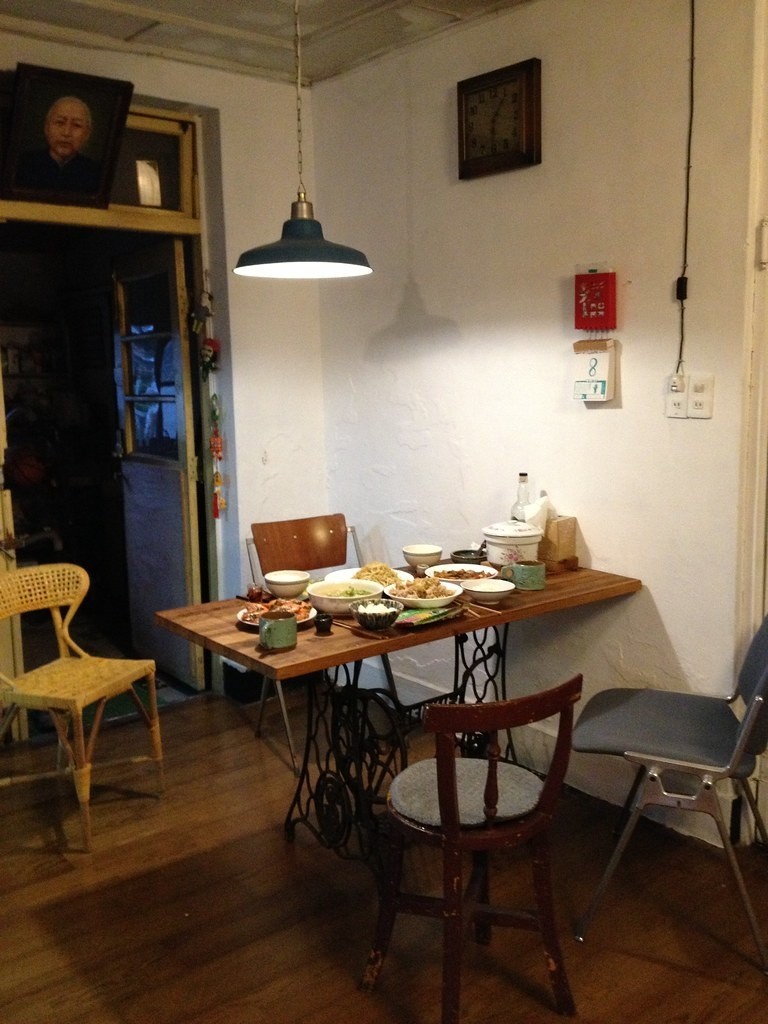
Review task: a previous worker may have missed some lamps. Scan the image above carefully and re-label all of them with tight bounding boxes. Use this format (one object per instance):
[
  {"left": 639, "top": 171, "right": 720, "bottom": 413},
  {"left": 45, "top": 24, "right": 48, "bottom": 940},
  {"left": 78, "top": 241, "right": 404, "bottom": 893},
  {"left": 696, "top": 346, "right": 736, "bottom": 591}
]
[{"left": 234, "top": 0, "right": 374, "bottom": 281}]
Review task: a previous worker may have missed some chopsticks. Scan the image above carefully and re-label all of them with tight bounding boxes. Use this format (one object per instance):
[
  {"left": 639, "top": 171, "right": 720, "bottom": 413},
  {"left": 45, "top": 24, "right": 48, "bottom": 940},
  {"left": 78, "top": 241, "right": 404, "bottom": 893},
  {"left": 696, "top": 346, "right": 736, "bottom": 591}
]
[
  {"left": 453, "top": 599, "right": 502, "bottom": 618},
  {"left": 332, "top": 619, "right": 390, "bottom": 640}
]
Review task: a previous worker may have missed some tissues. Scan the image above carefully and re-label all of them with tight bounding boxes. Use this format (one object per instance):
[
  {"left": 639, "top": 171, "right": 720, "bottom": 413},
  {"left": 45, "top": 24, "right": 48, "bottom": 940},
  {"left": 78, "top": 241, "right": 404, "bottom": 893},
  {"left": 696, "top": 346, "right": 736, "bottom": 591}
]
[{"left": 516, "top": 491, "right": 577, "bottom": 561}]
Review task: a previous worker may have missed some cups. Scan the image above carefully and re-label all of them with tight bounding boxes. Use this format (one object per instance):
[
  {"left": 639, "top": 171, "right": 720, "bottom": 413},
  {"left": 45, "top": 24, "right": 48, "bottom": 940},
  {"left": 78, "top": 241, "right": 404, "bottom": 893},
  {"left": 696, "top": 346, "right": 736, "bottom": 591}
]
[
  {"left": 246, "top": 583, "right": 263, "bottom": 602},
  {"left": 259, "top": 611, "right": 297, "bottom": 649},
  {"left": 501, "top": 560, "right": 546, "bottom": 591},
  {"left": 314, "top": 614, "right": 333, "bottom": 634}
]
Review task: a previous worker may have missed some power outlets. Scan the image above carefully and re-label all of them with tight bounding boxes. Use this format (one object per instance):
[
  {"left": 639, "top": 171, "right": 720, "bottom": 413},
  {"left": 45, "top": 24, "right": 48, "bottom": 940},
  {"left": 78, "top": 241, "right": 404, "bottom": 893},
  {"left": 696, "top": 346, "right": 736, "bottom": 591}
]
[{"left": 666, "top": 374, "right": 715, "bottom": 419}]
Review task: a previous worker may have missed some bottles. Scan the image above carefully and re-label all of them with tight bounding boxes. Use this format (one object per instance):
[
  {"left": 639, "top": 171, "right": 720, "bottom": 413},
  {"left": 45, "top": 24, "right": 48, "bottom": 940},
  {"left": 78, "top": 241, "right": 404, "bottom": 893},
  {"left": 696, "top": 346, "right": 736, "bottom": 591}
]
[{"left": 510, "top": 472, "right": 530, "bottom": 522}]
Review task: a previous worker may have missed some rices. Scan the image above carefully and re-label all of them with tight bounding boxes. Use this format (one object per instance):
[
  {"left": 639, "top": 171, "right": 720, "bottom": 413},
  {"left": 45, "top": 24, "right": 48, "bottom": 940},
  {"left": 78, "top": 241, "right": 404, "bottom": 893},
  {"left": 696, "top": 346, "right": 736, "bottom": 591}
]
[{"left": 356, "top": 604, "right": 397, "bottom": 612}]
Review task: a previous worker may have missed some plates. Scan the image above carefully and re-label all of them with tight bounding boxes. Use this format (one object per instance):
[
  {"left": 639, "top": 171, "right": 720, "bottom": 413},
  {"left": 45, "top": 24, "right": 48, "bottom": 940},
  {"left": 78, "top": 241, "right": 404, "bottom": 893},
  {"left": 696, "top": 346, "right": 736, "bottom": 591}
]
[
  {"left": 425, "top": 564, "right": 497, "bottom": 585},
  {"left": 238, "top": 607, "right": 318, "bottom": 626},
  {"left": 325, "top": 568, "right": 413, "bottom": 583}
]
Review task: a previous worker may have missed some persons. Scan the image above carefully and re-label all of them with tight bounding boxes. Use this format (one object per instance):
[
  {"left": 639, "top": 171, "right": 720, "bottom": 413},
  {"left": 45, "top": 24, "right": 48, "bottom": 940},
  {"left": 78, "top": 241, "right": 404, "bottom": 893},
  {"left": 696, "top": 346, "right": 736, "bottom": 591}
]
[{"left": 14, "top": 93, "right": 102, "bottom": 196}]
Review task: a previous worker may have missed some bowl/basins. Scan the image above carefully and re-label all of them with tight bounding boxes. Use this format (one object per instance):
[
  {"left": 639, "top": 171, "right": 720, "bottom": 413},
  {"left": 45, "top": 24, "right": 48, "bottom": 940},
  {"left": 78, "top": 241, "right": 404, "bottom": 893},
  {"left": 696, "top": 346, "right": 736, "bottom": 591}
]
[
  {"left": 264, "top": 570, "right": 310, "bottom": 601},
  {"left": 460, "top": 579, "right": 515, "bottom": 605},
  {"left": 402, "top": 544, "right": 442, "bottom": 571},
  {"left": 349, "top": 598, "right": 404, "bottom": 630},
  {"left": 307, "top": 580, "right": 384, "bottom": 615},
  {"left": 450, "top": 549, "right": 488, "bottom": 564},
  {"left": 384, "top": 581, "right": 462, "bottom": 608}
]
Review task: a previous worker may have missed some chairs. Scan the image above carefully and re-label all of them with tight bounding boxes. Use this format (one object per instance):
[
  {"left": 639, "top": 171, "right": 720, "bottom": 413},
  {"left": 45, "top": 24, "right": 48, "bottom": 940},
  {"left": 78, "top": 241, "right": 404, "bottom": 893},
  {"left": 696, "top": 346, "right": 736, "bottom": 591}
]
[
  {"left": 0, "top": 563, "right": 164, "bottom": 854},
  {"left": 569, "top": 615, "right": 768, "bottom": 976},
  {"left": 246, "top": 513, "right": 409, "bottom": 779},
  {"left": 360, "top": 673, "right": 577, "bottom": 1024}
]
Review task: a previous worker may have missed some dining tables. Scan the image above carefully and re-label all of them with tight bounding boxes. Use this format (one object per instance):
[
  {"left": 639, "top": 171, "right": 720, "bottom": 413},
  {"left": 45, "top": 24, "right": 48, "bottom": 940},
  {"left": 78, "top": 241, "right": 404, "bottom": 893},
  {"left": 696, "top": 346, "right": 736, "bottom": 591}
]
[{"left": 154, "top": 558, "right": 642, "bottom": 896}]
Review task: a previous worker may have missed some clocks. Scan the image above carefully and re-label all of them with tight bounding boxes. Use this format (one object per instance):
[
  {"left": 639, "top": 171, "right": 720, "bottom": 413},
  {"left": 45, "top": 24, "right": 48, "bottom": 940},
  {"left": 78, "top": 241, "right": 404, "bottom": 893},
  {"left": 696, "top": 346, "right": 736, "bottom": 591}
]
[{"left": 456, "top": 57, "right": 542, "bottom": 181}]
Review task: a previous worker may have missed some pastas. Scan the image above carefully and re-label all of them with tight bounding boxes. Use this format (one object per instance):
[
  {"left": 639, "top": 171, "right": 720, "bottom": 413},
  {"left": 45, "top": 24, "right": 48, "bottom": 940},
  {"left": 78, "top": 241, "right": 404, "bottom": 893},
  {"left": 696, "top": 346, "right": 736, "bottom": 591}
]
[{"left": 352, "top": 562, "right": 403, "bottom": 586}]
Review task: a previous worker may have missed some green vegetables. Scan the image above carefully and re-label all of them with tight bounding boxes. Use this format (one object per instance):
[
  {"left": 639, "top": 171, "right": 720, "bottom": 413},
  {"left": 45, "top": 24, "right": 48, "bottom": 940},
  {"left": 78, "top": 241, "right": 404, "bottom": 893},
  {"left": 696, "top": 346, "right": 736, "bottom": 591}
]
[{"left": 343, "top": 585, "right": 374, "bottom": 597}]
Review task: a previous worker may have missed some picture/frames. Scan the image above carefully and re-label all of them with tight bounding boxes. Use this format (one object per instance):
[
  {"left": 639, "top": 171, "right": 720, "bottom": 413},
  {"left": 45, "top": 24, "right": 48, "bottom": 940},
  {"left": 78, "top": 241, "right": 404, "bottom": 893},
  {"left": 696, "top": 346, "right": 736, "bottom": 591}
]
[{"left": 1, "top": 63, "right": 134, "bottom": 210}]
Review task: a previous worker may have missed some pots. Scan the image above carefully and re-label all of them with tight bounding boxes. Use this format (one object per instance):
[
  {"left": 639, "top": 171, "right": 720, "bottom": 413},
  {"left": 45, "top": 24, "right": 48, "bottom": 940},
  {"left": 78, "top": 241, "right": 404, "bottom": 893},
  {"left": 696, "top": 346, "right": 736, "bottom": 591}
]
[{"left": 482, "top": 521, "right": 544, "bottom": 567}]
